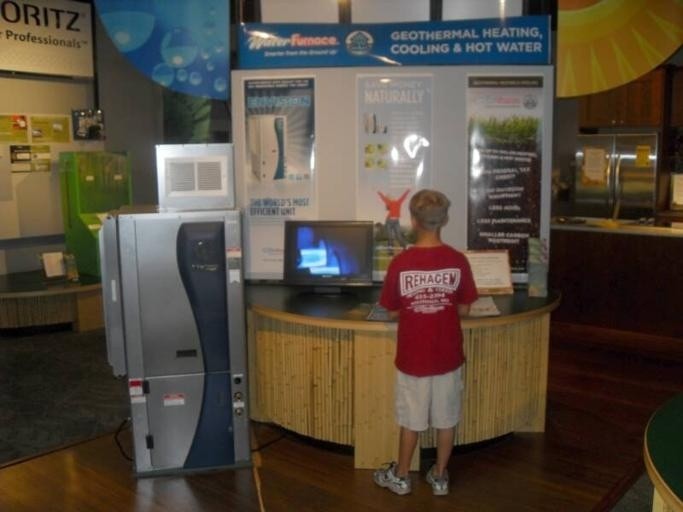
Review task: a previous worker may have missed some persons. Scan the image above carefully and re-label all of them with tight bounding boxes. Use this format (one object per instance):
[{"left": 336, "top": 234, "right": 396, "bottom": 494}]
[
  {"left": 371, "top": 190, "right": 478, "bottom": 498},
  {"left": 375, "top": 187, "right": 411, "bottom": 257}
]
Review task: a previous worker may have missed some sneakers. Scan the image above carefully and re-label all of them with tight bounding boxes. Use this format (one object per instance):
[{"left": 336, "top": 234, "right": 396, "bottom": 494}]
[
  {"left": 372, "top": 463, "right": 413, "bottom": 495},
  {"left": 425, "top": 464, "right": 450, "bottom": 496}
]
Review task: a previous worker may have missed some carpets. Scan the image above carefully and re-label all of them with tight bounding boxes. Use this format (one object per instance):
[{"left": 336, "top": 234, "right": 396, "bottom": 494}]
[{"left": 0, "top": 327, "right": 132, "bottom": 468}]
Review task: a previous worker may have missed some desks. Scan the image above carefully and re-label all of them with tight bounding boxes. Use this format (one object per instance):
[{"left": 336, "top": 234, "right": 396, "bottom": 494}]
[{"left": 245, "top": 284, "right": 563, "bottom": 467}]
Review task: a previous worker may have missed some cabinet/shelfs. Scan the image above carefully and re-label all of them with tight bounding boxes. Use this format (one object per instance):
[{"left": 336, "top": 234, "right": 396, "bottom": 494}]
[{"left": 577, "top": 63, "right": 670, "bottom": 129}]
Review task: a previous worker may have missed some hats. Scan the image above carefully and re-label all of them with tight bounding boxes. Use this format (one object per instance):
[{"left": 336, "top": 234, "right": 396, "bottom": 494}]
[{"left": 409, "top": 190, "right": 451, "bottom": 230}]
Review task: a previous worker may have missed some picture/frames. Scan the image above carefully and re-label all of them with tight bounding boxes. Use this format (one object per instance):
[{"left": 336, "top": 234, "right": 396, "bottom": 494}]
[{"left": 40, "top": 250, "right": 66, "bottom": 281}]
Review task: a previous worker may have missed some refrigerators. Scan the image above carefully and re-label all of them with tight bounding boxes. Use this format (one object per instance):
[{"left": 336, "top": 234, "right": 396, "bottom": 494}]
[{"left": 574, "top": 126, "right": 658, "bottom": 220}]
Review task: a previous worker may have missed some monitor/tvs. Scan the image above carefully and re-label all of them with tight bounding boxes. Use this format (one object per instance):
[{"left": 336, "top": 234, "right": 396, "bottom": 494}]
[{"left": 282, "top": 220, "right": 374, "bottom": 297}]
